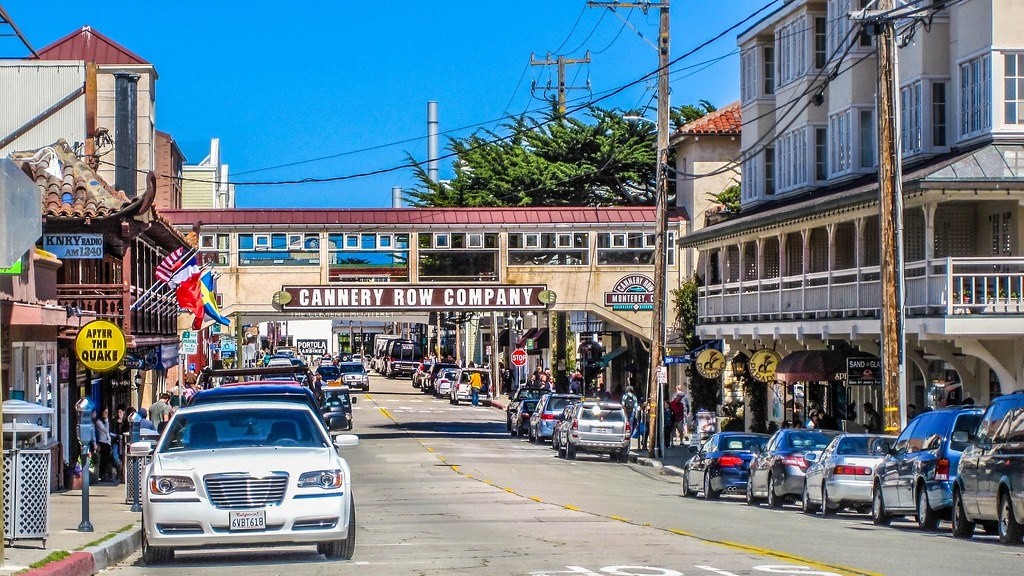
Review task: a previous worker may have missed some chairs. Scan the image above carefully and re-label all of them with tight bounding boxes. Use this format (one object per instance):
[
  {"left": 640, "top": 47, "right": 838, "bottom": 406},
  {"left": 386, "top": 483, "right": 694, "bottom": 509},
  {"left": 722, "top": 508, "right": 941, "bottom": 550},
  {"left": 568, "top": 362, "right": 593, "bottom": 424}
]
[
  {"left": 185, "top": 422, "right": 218, "bottom": 450},
  {"left": 268, "top": 420, "right": 299, "bottom": 444}
]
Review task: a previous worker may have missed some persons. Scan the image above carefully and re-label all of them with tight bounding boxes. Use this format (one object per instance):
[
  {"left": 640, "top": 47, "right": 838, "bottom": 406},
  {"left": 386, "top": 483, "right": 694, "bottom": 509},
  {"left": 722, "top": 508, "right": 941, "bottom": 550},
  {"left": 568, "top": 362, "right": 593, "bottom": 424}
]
[
  {"left": 424, "top": 353, "right": 691, "bottom": 450},
  {"left": 747, "top": 396, "right": 975, "bottom": 435},
  {"left": 96, "top": 347, "right": 272, "bottom": 481}
]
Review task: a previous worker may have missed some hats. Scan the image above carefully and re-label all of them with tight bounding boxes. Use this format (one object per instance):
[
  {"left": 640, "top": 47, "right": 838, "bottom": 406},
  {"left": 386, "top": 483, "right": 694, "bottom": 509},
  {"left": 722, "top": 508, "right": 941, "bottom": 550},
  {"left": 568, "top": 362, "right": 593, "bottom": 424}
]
[
  {"left": 138, "top": 408, "right": 147, "bottom": 418},
  {"left": 815, "top": 405, "right": 825, "bottom": 413},
  {"left": 809, "top": 409, "right": 818, "bottom": 418}
]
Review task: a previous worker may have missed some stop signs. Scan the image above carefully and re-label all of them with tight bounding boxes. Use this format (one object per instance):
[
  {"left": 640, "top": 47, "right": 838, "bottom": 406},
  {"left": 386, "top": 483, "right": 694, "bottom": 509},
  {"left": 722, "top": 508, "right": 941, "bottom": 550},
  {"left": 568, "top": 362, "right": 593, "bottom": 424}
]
[{"left": 511, "top": 349, "right": 527, "bottom": 366}]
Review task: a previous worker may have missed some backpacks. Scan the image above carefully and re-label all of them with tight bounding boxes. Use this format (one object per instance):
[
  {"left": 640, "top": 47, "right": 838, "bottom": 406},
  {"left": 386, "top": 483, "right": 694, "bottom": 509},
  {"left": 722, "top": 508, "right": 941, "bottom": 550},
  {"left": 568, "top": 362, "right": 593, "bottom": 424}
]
[{"left": 624, "top": 394, "right": 635, "bottom": 410}]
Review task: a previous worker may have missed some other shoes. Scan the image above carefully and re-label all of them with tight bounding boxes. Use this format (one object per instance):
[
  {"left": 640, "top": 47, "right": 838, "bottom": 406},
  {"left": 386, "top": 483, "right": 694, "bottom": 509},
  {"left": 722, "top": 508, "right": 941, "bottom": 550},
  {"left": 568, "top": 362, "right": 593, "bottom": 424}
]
[
  {"left": 101, "top": 477, "right": 114, "bottom": 482},
  {"left": 469, "top": 404, "right": 475, "bottom": 407},
  {"left": 476, "top": 405, "right": 480, "bottom": 407},
  {"left": 671, "top": 443, "right": 676, "bottom": 448},
  {"left": 680, "top": 444, "right": 688, "bottom": 448}
]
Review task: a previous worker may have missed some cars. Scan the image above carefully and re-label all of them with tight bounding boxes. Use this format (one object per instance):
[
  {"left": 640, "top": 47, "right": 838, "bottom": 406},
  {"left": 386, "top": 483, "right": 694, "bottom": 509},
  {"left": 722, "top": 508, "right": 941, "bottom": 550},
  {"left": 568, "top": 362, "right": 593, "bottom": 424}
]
[
  {"left": 872, "top": 404, "right": 985, "bottom": 532},
  {"left": 421, "top": 362, "right": 460, "bottom": 395},
  {"left": 157, "top": 354, "right": 348, "bottom": 447},
  {"left": 351, "top": 354, "right": 366, "bottom": 362},
  {"left": 506, "top": 387, "right": 585, "bottom": 445},
  {"left": 802, "top": 433, "right": 912, "bottom": 518},
  {"left": 552, "top": 398, "right": 631, "bottom": 463},
  {"left": 746, "top": 428, "right": 856, "bottom": 509},
  {"left": 412, "top": 363, "right": 431, "bottom": 389},
  {"left": 319, "top": 386, "right": 357, "bottom": 429},
  {"left": 130, "top": 401, "right": 358, "bottom": 566},
  {"left": 433, "top": 368, "right": 470, "bottom": 398},
  {"left": 683, "top": 432, "right": 773, "bottom": 501}
]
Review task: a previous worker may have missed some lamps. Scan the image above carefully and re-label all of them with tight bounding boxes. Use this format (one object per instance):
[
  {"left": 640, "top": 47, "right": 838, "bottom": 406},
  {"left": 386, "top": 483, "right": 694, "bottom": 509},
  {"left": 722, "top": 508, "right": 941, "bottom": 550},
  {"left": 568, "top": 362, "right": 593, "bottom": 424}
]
[
  {"left": 66, "top": 304, "right": 73, "bottom": 318},
  {"left": 21, "top": 146, "right": 62, "bottom": 181},
  {"left": 74, "top": 306, "right": 84, "bottom": 317},
  {"left": 129, "top": 371, "right": 142, "bottom": 393}
]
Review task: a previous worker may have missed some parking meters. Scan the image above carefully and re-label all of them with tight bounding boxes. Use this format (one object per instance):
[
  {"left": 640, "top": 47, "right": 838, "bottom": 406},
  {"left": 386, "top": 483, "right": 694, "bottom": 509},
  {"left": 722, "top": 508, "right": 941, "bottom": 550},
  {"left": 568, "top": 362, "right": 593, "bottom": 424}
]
[
  {"left": 75, "top": 397, "right": 95, "bottom": 532},
  {"left": 128, "top": 412, "right": 143, "bottom": 512}
]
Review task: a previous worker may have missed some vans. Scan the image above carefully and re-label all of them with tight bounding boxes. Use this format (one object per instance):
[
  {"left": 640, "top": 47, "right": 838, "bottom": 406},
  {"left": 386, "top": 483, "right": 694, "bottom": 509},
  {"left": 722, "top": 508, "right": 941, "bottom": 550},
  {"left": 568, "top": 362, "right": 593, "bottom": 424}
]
[
  {"left": 448, "top": 368, "right": 494, "bottom": 407},
  {"left": 371, "top": 335, "right": 422, "bottom": 379}
]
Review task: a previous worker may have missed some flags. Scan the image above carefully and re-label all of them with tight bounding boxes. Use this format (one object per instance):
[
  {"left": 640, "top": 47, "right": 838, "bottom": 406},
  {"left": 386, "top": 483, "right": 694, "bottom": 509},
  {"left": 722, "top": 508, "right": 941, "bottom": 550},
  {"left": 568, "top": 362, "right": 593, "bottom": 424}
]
[{"left": 154, "top": 246, "right": 231, "bottom": 331}]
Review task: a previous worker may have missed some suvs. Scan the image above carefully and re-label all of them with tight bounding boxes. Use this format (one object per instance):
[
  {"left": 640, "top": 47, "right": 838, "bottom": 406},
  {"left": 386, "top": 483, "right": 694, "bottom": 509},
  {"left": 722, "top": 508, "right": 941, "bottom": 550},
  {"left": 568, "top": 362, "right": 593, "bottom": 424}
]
[
  {"left": 950, "top": 389, "right": 1024, "bottom": 545},
  {"left": 339, "top": 362, "right": 371, "bottom": 391}
]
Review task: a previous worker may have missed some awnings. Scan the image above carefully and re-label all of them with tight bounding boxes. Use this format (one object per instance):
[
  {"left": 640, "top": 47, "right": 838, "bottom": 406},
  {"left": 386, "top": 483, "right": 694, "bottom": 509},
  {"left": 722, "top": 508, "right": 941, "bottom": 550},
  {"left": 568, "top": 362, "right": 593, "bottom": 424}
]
[
  {"left": 775, "top": 350, "right": 859, "bottom": 383},
  {"left": 493, "top": 327, "right": 549, "bottom": 349},
  {"left": 600, "top": 346, "right": 629, "bottom": 366}
]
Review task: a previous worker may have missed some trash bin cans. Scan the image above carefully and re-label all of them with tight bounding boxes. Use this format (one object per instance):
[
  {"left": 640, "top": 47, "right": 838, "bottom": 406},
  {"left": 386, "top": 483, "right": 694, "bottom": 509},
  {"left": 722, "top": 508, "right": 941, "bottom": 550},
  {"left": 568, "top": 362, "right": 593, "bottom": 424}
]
[
  {"left": 123, "top": 427, "right": 160, "bottom": 504},
  {"left": 2, "top": 399, "right": 54, "bottom": 549}
]
